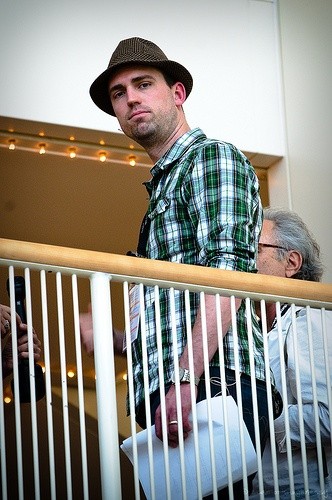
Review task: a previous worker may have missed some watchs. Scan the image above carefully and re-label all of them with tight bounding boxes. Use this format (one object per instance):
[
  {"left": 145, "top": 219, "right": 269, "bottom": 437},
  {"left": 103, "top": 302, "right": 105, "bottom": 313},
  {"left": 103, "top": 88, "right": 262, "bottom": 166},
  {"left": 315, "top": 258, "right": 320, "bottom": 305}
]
[{"left": 167, "top": 368, "right": 199, "bottom": 387}]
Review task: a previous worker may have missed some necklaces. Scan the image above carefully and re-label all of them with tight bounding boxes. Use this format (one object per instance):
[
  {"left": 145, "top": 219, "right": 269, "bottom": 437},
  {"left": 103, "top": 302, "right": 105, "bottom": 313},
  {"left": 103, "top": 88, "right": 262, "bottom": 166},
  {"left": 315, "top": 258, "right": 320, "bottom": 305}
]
[{"left": 79, "top": 37, "right": 283, "bottom": 500}]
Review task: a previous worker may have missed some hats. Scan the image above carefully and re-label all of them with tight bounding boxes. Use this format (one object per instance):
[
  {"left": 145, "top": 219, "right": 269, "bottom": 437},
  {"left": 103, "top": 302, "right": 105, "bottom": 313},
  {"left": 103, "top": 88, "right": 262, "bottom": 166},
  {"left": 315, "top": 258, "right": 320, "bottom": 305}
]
[{"left": 91, "top": 37, "right": 194, "bottom": 117}]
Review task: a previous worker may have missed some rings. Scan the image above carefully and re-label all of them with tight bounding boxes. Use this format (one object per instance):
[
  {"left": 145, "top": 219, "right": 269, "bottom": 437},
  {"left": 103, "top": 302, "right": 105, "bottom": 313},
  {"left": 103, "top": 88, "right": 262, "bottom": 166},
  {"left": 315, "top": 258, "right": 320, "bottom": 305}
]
[
  {"left": 4, "top": 320, "right": 9, "bottom": 328},
  {"left": 168, "top": 420, "right": 177, "bottom": 424}
]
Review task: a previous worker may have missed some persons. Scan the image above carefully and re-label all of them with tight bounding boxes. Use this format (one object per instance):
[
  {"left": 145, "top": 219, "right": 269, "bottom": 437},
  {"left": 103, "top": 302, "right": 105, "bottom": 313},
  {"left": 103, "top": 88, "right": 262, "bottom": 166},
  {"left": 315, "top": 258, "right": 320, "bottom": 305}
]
[
  {"left": 254, "top": 210, "right": 332, "bottom": 500},
  {"left": 0, "top": 303, "right": 42, "bottom": 379}
]
